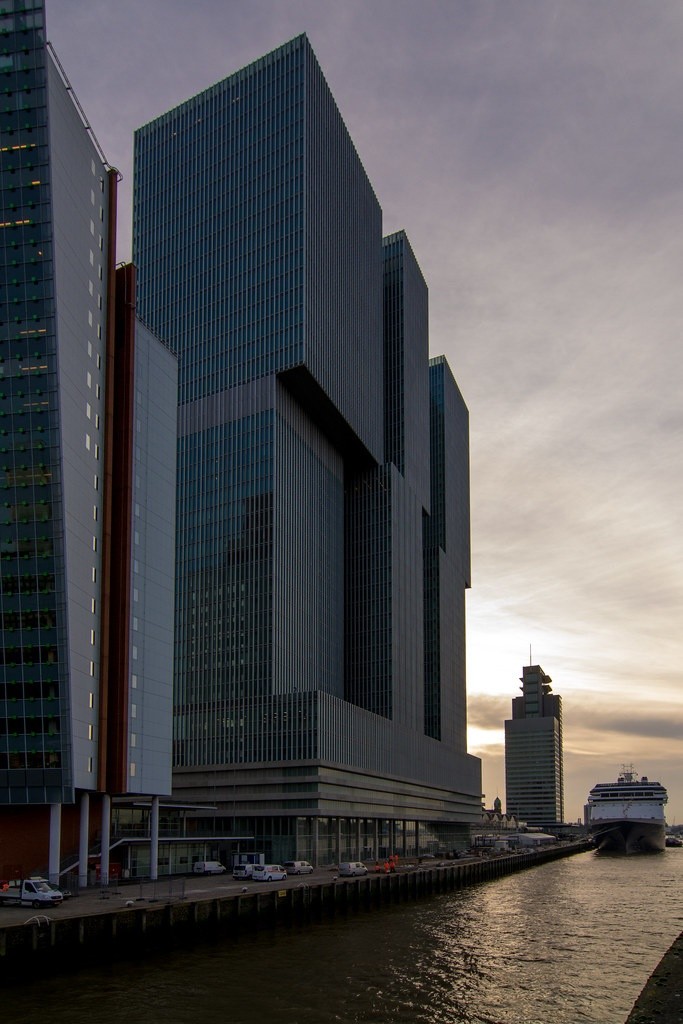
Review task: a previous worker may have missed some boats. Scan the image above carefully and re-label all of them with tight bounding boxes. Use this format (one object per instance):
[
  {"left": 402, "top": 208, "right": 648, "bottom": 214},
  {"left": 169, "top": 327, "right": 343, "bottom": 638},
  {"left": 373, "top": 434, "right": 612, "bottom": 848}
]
[
  {"left": 583, "top": 763, "right": 669, "bottom": 856},
  {"left": 665, "top": 837, "right": 683, "bottom": 847}
]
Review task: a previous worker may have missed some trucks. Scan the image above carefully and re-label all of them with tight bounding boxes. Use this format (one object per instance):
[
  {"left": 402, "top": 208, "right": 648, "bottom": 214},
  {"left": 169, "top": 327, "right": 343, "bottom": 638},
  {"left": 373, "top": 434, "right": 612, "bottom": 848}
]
[{"left": 1, "top": 878, "right": 64, "bottom": 910}]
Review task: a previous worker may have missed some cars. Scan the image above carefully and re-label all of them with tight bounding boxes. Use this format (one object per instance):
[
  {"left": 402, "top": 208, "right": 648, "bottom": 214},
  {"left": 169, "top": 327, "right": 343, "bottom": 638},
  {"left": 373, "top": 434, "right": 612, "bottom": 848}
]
[{"left": 50, "top": 883, "right": 72, "bottom": 900}]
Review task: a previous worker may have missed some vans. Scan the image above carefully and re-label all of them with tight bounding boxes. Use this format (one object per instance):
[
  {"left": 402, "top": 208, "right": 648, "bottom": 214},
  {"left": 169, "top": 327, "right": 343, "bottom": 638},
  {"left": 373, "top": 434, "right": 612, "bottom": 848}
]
[
  {"left": 338, "top": 861, "right": 369, "bottom": 877},
  {"left": 283, "top": 860, "right": 314, "bottom": 876},
  {"left": 252, "top": 864, "right": 288, "bottom": 882},
  {"left": 233, "top": 863, "right": 257, "bottom": 881},
  {"left": 193, "top": 861, "right": 227, "bottom": 877}
]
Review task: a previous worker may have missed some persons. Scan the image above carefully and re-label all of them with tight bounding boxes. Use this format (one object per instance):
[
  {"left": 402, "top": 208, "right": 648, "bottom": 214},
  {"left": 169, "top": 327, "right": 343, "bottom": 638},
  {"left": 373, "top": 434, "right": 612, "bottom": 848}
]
[{"left": 375, "top": 853, "right": 399, "bottom": 874}]
